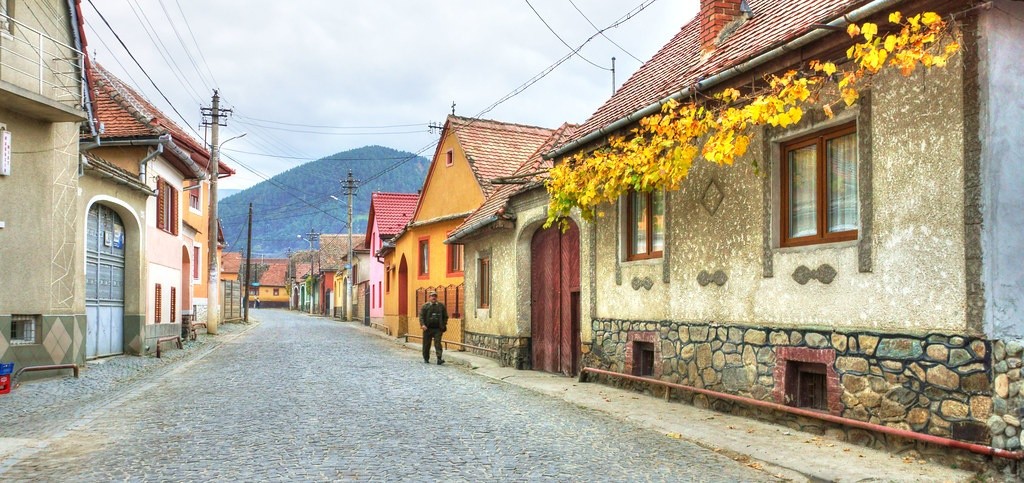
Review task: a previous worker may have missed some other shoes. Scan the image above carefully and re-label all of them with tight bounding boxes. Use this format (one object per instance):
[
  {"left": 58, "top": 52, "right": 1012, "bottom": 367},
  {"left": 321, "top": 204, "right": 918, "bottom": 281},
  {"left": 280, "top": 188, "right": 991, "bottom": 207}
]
[
  {"left": 425, "top": 360, "right": 429, "bottom": 363},
  {"left": 437, "top": 360, "right": 445, "bottom": 365}
]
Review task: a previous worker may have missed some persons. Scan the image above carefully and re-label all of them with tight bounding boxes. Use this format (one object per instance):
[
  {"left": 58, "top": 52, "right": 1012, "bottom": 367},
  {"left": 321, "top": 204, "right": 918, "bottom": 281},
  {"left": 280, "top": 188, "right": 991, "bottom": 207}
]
[{"left": 418, "top": 290, "right": 449, "bottom": 365}]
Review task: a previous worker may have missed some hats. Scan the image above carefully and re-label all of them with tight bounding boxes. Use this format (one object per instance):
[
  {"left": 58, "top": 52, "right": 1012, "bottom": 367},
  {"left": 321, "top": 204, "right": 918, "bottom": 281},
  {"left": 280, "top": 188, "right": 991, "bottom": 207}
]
[{"left": 428, "top": 291, "right": 437, "bottom": 296}]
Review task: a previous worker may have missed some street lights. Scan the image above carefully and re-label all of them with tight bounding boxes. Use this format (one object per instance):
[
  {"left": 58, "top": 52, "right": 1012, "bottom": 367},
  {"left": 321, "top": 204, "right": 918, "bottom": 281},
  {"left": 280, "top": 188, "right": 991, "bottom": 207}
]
[
  {"left": 208, "top": 131, "right": 249, "bottom": 335},
  {"left": 297, "top": 233, "right": 315, "bottom": 315},
  {"left": 328, "top": 193, "right": 354, "bottom": 322}
]
